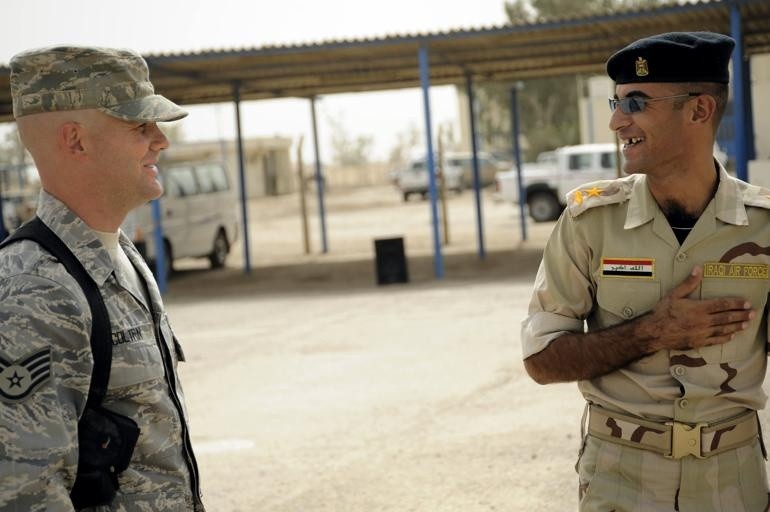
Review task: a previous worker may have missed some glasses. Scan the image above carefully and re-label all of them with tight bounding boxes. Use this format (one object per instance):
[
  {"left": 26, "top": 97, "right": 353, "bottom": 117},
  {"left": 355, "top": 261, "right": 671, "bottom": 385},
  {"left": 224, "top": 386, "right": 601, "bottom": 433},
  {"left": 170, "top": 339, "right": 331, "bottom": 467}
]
[{"left": 607, "top": 92, "right": 703, "bottom": 116}]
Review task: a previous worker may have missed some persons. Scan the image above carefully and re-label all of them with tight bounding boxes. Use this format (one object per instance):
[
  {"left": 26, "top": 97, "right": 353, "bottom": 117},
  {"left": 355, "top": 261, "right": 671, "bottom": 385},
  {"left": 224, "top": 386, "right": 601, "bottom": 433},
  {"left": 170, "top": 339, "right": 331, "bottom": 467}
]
[
  {"left": 520, "top": 30, "right": 770, "bottom": 512},
  {"left": 0, "top": 45, "right": 205, "bottom": 512}
]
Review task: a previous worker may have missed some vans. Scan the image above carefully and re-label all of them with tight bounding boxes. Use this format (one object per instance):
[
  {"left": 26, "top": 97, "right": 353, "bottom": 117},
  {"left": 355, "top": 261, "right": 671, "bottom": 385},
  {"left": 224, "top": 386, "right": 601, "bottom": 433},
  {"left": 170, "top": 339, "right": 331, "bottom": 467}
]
[
  {"left": 0, "top": 192, "right": 34, "bottom": 226},
  {"left": 34, "top": 143, "right": 240, "bottom": 287}
]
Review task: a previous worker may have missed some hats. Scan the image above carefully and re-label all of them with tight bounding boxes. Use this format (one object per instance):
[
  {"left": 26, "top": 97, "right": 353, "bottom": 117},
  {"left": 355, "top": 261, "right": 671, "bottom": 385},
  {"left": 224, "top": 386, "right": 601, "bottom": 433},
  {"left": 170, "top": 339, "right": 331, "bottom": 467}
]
[
  {"left": 605, "top": 31, "right": 735, "bottom": 84},
  {"left": 9, "top": 45, "right": 188, "bottom": 123}
]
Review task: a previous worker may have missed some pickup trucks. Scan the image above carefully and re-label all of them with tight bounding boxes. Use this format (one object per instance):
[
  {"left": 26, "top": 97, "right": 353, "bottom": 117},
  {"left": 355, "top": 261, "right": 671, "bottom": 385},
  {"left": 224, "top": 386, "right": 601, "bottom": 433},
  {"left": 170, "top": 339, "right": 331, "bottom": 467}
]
[{"left": 491, "top": 144, "right": 728, "bottom": 222}]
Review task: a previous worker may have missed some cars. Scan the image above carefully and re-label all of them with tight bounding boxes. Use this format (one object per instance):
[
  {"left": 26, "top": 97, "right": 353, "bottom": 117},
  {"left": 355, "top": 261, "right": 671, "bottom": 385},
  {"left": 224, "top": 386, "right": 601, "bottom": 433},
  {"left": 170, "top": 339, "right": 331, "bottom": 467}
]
[
  {"left": 535, "top": 150, "right": 559, "bottom": 165},
  {"left": 393, "top": 150, "right": 511, "bottom": 202}
]
